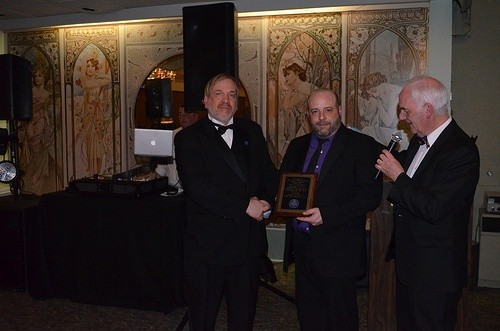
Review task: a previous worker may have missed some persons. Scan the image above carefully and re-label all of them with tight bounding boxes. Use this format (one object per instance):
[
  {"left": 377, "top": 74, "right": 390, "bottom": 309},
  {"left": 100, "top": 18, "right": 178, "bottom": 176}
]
[
  {"left": 375, "top": 76, "right": 479, "bottom": 331},
  {"left": 174, "top": 74, "right": 279, "bottom": 330},
  {"left": 260, "top": 89, "right": 384, "bottom": 331}
]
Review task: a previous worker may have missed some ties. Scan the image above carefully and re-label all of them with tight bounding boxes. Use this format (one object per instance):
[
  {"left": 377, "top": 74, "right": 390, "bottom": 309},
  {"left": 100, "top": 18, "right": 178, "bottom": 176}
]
[{"left": 297, "top": 136, "right": 330, "bottom": 225}]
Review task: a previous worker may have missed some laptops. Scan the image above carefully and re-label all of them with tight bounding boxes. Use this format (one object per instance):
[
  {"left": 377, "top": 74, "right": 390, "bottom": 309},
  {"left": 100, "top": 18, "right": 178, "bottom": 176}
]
[{"left": 135, "top": 129, "right": 174, "bottom": 157}]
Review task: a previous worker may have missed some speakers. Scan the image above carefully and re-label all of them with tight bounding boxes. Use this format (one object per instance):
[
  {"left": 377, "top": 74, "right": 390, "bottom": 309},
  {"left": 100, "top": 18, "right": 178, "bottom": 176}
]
[
  {"left": 182, "top": 2, "right": 239, "bottom": 113},
  {"left": 145, "top": 77, "right": 172, "bottom": 118},
  {"left": 0, "top": 54, "right": 33, "bottom": 120}
]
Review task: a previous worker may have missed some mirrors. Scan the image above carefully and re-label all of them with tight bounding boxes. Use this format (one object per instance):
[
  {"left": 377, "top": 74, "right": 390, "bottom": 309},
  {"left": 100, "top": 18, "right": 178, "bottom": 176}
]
[{"left": 133, "top": 51, "right": 251, "bottom": 164}]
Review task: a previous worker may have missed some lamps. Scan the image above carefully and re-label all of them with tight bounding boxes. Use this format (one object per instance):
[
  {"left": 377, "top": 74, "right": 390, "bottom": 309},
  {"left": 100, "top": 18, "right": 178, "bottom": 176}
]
[{"left": 133, "top": 126, "right": 174, "bottom": 172}]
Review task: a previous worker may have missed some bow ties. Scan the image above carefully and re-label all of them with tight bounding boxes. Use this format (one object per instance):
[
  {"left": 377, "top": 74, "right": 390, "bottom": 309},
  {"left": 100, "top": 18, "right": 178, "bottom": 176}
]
[
  {"left": 213, "top": 122, "right": 235, "bottom": 136},
  {"left": 414, "top": 137, "right": 430, "bottom": 149}
]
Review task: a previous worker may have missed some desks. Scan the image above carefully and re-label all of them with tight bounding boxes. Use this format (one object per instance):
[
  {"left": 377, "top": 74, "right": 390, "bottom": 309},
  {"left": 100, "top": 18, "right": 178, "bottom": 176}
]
[{"left": 39, "top": 190, "right": 185, "bottom": 314}]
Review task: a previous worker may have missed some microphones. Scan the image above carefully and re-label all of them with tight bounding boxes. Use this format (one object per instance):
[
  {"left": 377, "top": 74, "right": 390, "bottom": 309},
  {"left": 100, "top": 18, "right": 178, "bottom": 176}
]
[{"left": 375, "top": 131, "right": 403, "bottom": 180}]
